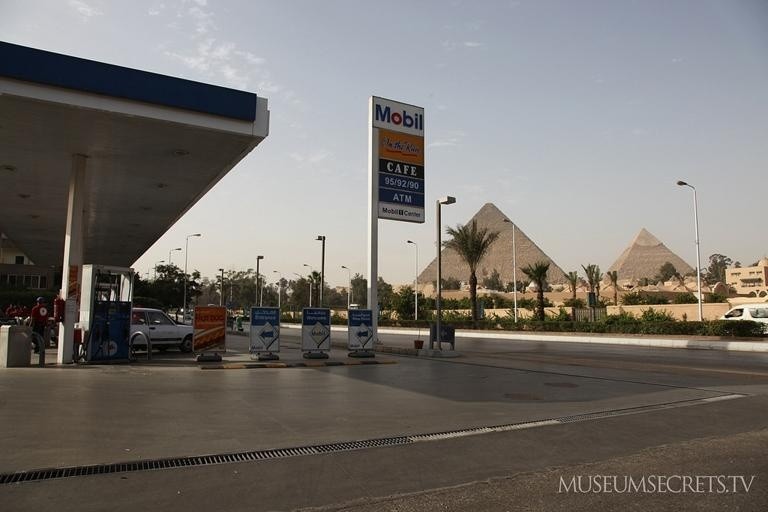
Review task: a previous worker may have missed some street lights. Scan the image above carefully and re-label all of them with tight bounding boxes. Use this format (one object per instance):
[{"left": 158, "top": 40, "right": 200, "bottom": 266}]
[
  {"left": 143, "top": 234, "right": 351, "bottom": 325},
  {"left": 435, "top": 196, "right": 456, "bottom": 351},
  {"left": 678, "top": 180, "right": 703, "bottom": 321},
  {"left": 408, "top": 241, "right": 418, "bottom": 321},
  {"left": 503, "top": 219, "right": 517, "bottom": 323}
]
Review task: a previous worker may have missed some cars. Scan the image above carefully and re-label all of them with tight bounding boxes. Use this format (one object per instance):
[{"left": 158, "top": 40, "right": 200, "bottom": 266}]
[
  {"left": 719, "top": 304, "right": 768, "bottom": 334},
  {"left": 131, "top": 307, "right": 194, "bottom": 353}
]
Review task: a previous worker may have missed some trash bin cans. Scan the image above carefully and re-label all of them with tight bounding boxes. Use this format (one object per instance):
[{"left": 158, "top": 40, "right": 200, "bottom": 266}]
[{"left": 1, "top": 325, "right": 32, "bottom": 366}]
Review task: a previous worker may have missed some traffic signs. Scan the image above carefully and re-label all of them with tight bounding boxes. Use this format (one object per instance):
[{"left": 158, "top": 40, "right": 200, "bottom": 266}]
[
  {"left": 347, "top": 309, "right": 374, "bottom": 350},
  {"left": 250, "top": 307, "right": 280, "bottom": 353},
  {"left": 302, "top": 308, "right": 330, "bottom": 351}
]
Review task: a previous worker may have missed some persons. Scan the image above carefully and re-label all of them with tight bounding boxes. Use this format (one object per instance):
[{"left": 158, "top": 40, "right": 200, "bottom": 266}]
[
  {"left": 29, "top": 296, "right": 50, "bottom": 353},
  {"left": 4, "top": 303, "right": 29, "bottom": 322}
]
